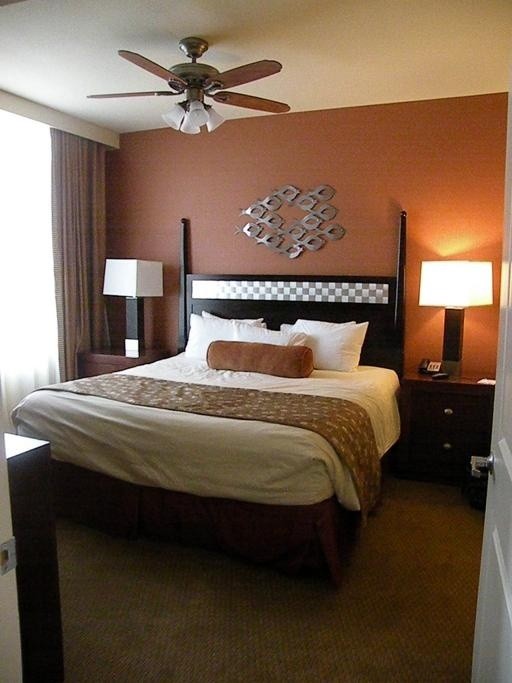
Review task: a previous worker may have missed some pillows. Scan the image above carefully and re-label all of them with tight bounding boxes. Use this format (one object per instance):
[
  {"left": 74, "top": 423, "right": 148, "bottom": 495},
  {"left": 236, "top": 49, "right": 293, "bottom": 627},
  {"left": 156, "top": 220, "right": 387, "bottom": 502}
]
[
  {"left": 200, "top": 311, "right": 263, "bottom": 328},
  {"left": 287, "top": 319, "right": 370, "bottom": 374},
  {"left": 293, "top": 319, "right": 356, "bottom": 327},
  {"left": 232, "top": 320, "right": 307, "bottom": 348},
  {"left": 206, "top": 340, "right": 315, "bottom": 379},
  {"left": 184, "top": 313, "right": 263, "bottom": 361}
]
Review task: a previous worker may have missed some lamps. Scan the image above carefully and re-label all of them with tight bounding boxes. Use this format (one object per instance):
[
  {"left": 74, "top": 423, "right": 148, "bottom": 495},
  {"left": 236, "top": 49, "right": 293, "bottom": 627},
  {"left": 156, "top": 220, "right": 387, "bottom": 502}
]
[
  {"left": 102, "top": 258, "right": 164, "bottom": 351},
  {"left": 417, "top": 259, "right": 495, "bottom": 373},
  {"left": 161, "top": 99, "right": 187, "bottom": 135},
  {"left": 188, "top": 98, "right": 209, "bottom": 128},
  {"left": 180, "top": 109, "right": 202, "bottom": 136},
  {"left": 204, "top": 104, "right": 230, "bottom": 134}
]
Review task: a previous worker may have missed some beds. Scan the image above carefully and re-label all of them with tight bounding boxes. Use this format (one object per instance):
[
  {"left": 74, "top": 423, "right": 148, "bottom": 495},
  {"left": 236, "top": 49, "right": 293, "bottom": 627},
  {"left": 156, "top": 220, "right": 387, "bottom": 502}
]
[{"left": 10, "top": 210, "right": 409, "bottom": 592}]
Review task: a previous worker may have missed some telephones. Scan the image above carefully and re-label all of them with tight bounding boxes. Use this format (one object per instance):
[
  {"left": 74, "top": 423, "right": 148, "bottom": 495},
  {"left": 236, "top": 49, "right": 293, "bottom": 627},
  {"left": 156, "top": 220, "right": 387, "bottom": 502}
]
[{"left": 418, "top": 358, "right": 448, "bottom": 376}]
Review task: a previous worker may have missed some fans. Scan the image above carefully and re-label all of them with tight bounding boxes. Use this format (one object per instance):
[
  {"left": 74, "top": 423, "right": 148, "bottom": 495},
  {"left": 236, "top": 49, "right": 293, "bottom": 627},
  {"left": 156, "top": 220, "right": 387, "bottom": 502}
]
[{"left": 87, "top": 33, "right": 292, "bottom": 115}]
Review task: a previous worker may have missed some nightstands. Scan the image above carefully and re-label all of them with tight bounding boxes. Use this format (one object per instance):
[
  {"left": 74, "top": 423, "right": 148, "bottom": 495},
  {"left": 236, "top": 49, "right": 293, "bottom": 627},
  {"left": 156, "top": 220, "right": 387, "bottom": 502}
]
[
  {"left": 77, "top": 347, "right": 173, "bottom": 379},
  {"left": 387, "top": 367, "right": 495, "bottom": 489}
]
